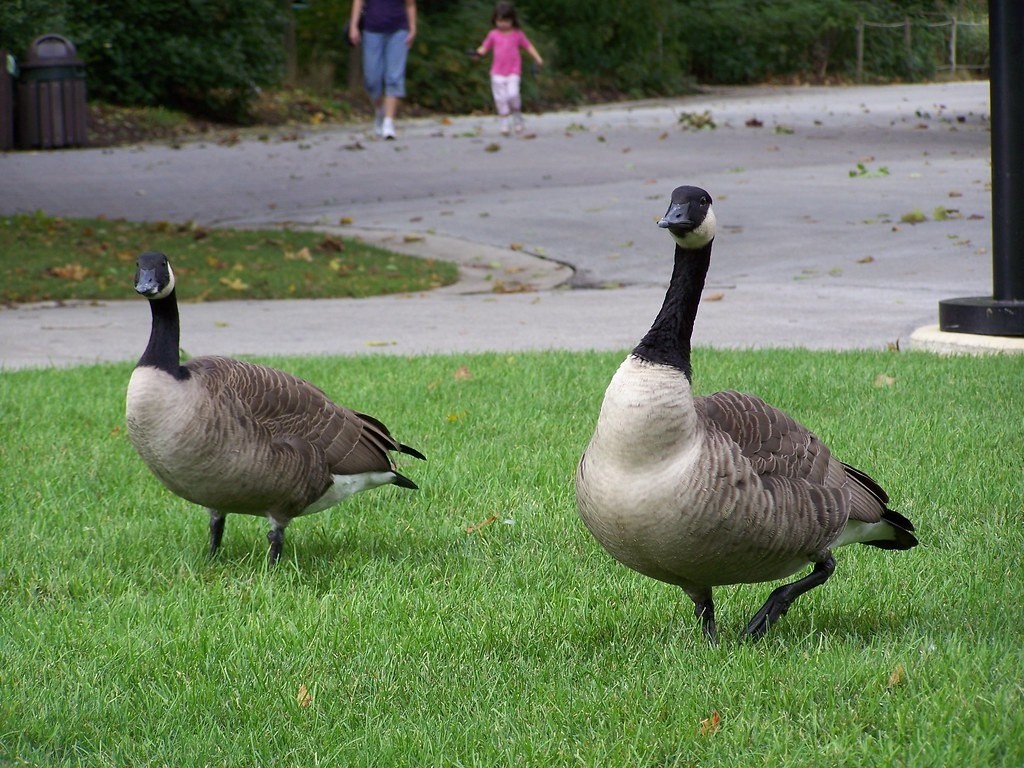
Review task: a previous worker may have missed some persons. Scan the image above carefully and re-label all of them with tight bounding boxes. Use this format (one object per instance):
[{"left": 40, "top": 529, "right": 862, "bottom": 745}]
[
  {"left": 473, "top": 3, "right": 544, "bottom": 135},
  {"left": 349, "top": 0, "right": 416, "bottom": 140}
]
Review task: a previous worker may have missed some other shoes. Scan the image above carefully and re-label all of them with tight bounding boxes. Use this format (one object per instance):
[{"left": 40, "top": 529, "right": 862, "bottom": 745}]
[
  {"left": 514, "top": 120, "right": 523, "bottom": 134},
  {"left": 382, "top": 125, "right": 395, "bottom": 140},
  {"left": 375, "top": 118, "right": 382, "bottom": 135},
  {"left": 501, "top": 122, "right": 511, "bottom": 134}
]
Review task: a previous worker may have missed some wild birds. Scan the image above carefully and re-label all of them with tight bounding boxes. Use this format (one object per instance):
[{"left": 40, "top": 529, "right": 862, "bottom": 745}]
[
  {"left": 124, "top": 250, "right": 427, "bottom": 580},
  {"left": 574, "top": 186, "right": 920, "bottom": 650}
]
